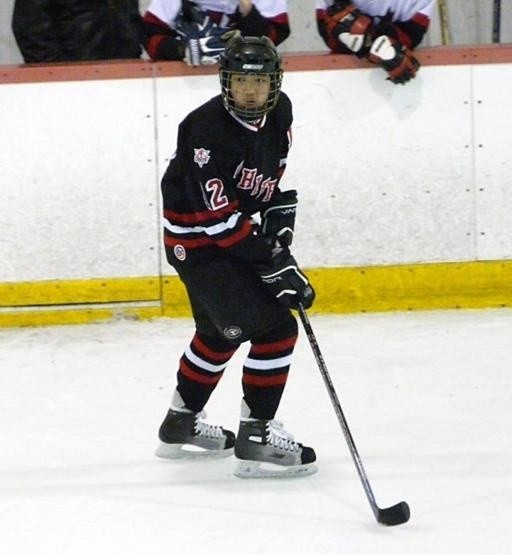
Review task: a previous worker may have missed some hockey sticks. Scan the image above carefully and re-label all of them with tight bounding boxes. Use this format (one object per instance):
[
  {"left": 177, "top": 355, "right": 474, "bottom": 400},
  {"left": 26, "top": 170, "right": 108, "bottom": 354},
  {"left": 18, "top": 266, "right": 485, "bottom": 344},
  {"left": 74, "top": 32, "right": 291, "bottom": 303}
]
[{"left": 297, "top": 301, "right": 410, "bottom": 525}]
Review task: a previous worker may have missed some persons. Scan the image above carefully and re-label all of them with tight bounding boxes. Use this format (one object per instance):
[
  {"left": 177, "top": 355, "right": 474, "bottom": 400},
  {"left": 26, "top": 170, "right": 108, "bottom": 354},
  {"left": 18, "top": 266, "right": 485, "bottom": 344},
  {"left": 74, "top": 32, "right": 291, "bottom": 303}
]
[
  {"left": 12, "top": 0, "right": 149, "bottom": 63},
  {"left": 143, "top": 0, "right": 290, "bottom": 67},
  {"left": 159, "top": 33, "right": 315, "bottom": 466},
  {"left": 315, "top": 0, "right": 435, "bottom": 85}
]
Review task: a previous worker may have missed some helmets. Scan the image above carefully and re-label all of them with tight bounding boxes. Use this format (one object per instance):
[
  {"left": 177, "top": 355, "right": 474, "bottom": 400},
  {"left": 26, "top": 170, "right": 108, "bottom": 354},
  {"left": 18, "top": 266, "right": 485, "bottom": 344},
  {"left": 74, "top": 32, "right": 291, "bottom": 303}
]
[{"left": 218, "top": 34, "right": 282, "bottom": 76}]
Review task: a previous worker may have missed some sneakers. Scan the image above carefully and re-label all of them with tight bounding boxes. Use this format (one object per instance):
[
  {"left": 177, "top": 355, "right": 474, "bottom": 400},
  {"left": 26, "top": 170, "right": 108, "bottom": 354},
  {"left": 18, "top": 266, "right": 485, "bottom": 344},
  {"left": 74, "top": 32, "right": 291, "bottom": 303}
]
[
  {"left": 158, "top": 407, "right": 235, "bottom": 451},
  {"left": 234, "top": 420, "right": 316, "bottom": 467}
]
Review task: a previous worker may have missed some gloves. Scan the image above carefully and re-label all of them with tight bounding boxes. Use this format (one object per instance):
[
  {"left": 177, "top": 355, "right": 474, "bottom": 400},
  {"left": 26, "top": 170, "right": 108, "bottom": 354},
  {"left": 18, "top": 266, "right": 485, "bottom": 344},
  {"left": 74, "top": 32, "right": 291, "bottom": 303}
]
[
  {"left": 315, "top": 0, "right": 431, "bottom": 85},
  {"left": 226, "top": 189, "right": 316, "bottom": 311},
  {"left": 173, "top": 0, "right": 242, "bottom": 68}
]
